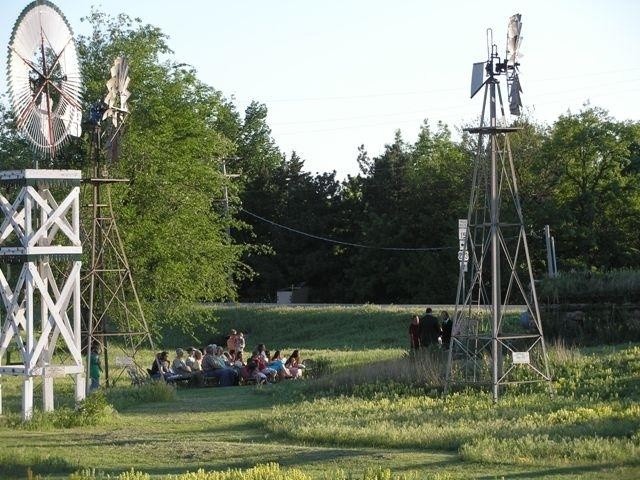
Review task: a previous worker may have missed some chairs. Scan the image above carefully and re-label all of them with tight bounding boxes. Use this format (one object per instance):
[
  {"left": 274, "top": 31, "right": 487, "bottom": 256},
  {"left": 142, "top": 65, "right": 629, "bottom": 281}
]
[{"left": 125, "top": 365, "right": 153, "bottom": 389}]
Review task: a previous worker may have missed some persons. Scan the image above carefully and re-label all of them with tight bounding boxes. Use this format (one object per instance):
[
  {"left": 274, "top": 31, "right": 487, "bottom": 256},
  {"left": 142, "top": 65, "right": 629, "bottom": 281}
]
[
  {"left": 418, "top": 308, "right": 442, "bottom": 348},
  {"left": 151, "top": 328, "right": 307, "bottom": 389},
  {"left": 410, "top": 314, "right": 422, "bottom": 350},
  {"left": 440, "top": 311, "right": 453, "bottom": 351},
  {"left": 86, "top": 344, "right": 104, "bottom": 397}
]
